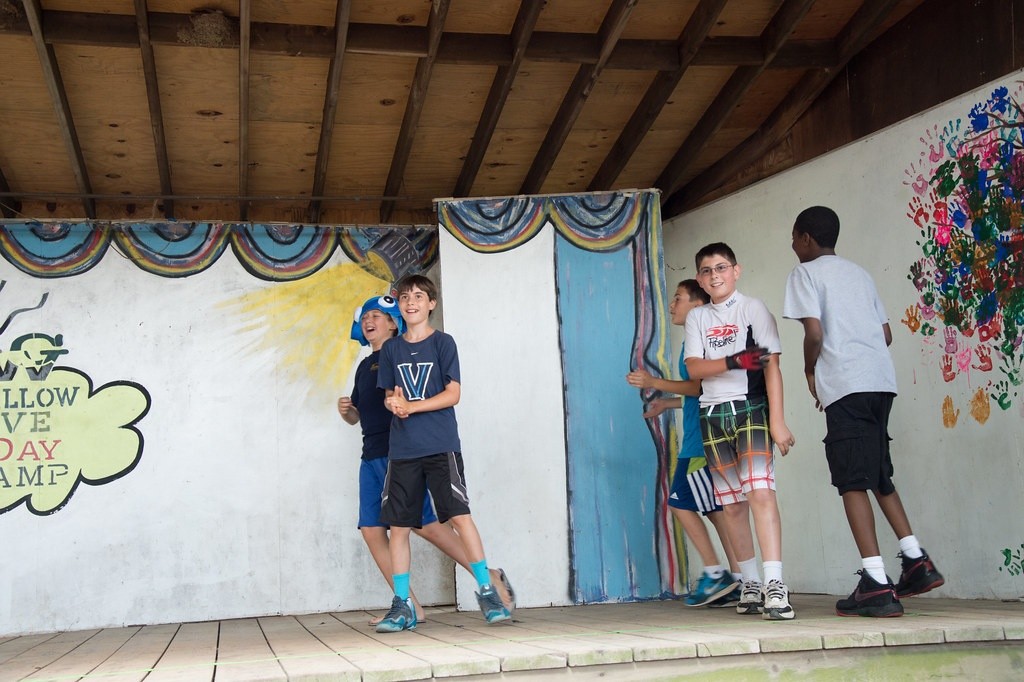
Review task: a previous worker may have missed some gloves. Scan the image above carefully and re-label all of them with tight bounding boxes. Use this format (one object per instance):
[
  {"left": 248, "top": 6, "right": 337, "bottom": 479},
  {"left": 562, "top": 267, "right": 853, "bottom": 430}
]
[{"left": 726, "top": 343, "right": 769, "bottom": 371}]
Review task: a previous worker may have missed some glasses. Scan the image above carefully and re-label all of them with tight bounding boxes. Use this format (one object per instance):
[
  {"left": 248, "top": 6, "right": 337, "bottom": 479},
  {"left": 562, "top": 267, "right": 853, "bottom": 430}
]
[{"left": 698, "top": 264, "right": 736, "bottom": 276}]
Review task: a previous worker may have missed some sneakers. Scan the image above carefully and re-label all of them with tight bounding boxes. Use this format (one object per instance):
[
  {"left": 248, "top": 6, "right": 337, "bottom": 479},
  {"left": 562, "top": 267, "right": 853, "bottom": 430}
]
[
  {"left": 894, "top": 548, "right": 945, "bottom": 597},
  {"left": 374, "top": 596, "right": 417, "bottom": 631},
  {"left": 835, "top": 568, "right": 903, "bottom": 618},
  {"left": 704, "top": 586, "right": 739, "bottom": 608},
  {"left": 683, "top": 570, "right": 739, "bottom": 607},
  {"left": 763, "top": 580, "right": 795, "bottom": 620},
  {"left": 737, "top": 581, "right": 764, "bottom": 614},
  {"left": 474, "top": 583, "right": 511, "bottom": 625}
]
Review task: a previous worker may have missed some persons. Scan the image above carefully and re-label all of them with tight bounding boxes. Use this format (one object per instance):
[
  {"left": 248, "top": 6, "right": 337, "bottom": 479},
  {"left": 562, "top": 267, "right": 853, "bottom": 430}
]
[
  {"left": 338, "top": 294, "right": 520, "bottom": 629},
  {"left": 779, "top": 205, "right": 948, "bottom": 619},
  {"left": 678, "top": 240, "right": 800, "bottom": 623},
  {"left": 626, "top": 278, "right": 752, "bottom": 612},
  {"left": 373, "top": 273, "right": 512, "bottom": 634}
]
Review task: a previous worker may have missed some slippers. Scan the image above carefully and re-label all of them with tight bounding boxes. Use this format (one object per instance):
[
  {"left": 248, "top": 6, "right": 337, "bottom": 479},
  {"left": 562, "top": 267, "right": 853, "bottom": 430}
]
[
  {"left": 497, "top": 568, "right": 515, "bottom": 612},
  {"left": 369, "top": 619, "right": 428, "bottom": 625}
]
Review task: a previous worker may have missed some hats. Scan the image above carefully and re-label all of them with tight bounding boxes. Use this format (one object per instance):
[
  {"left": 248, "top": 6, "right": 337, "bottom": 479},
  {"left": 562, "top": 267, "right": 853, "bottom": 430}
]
[{"left": 351, "top": 294, "right": 405, "bottom": 347}]
